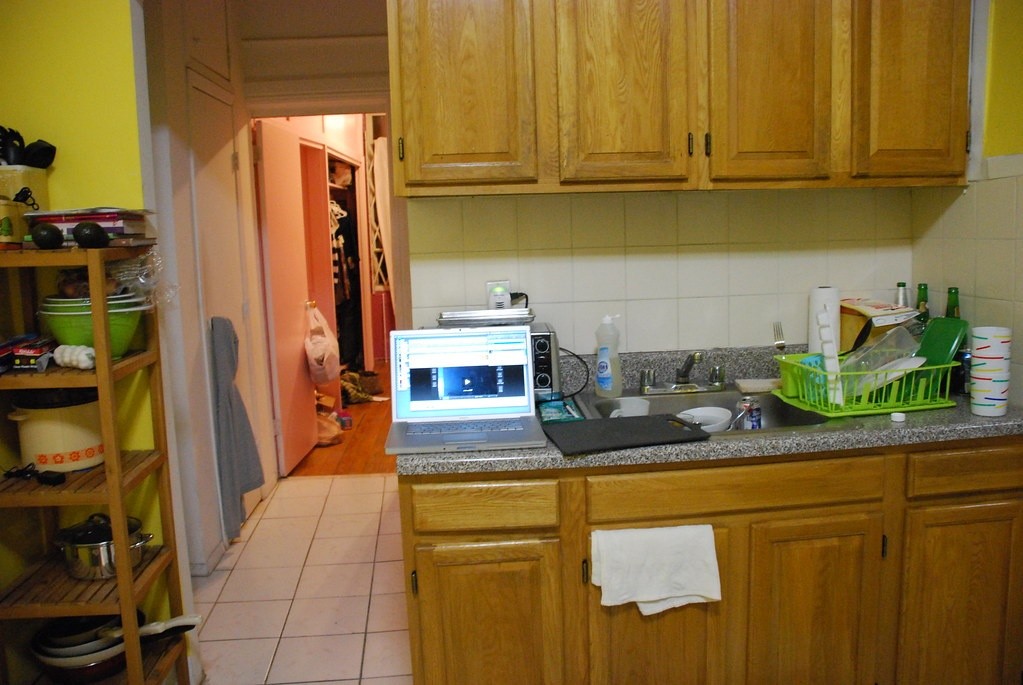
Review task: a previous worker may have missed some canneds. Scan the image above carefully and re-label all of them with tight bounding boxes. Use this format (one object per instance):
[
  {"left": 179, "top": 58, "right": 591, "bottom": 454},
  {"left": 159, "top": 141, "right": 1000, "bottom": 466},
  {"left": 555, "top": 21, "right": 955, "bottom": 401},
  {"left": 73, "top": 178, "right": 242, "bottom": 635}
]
[
  {"left": 735, "top": 396, "right": 761, "bottom": 430},
  {"left": 954, "top": 348, "right": 972, "bottom": 394}
]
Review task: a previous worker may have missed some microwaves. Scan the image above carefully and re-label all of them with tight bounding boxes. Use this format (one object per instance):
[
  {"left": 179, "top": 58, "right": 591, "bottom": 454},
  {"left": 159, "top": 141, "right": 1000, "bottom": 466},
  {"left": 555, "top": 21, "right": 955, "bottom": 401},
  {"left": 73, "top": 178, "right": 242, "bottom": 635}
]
[{"left": 417, "top": 322, "right": 559, "bottom": 402}]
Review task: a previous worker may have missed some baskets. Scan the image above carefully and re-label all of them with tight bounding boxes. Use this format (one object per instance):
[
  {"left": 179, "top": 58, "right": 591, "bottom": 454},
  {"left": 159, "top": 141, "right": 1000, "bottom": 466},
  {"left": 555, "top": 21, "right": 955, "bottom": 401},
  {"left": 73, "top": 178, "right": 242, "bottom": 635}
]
[{"left": 774, "top": 346, "right": 962, "bottom": 411}]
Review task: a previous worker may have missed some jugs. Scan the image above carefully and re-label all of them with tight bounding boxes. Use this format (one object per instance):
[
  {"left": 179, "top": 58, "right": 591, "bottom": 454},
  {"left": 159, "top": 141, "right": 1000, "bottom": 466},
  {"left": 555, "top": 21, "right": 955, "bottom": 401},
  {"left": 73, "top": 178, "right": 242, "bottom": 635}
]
[{"left": 608, "top": 397, "right": 650, "bottom": 419}]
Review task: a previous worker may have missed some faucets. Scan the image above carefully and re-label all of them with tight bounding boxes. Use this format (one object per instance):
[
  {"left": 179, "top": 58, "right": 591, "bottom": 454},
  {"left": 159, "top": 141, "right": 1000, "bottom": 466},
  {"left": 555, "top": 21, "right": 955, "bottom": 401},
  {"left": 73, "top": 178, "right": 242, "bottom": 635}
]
[{"left": 675, "top": 352, "right": 703, "bottom": 384}]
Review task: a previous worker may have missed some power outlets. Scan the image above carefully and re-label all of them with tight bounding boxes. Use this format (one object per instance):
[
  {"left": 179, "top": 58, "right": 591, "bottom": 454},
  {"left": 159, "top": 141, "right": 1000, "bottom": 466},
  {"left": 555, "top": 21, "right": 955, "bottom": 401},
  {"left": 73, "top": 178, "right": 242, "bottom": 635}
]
[{"left": 487, "top": 281, "right": 511, "bottom": 298}]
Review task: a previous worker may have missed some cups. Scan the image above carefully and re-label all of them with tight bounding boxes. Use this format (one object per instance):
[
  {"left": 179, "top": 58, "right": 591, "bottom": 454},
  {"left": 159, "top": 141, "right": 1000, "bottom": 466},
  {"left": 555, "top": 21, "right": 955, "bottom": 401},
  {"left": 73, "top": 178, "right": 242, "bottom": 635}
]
[
  {"left": 970, "top": 326, "right": 1013, "bottom": 416},
  {"left": 802, "top": 354, "right": 828, "bottom": 401}
]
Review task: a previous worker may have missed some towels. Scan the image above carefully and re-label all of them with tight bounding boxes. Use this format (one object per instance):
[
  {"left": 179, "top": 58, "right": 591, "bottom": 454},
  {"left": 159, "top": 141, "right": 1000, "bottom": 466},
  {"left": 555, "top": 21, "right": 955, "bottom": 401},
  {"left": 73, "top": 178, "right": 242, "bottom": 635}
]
[{"left": 591, "top": 524, "right": 722, "bottom": 617}]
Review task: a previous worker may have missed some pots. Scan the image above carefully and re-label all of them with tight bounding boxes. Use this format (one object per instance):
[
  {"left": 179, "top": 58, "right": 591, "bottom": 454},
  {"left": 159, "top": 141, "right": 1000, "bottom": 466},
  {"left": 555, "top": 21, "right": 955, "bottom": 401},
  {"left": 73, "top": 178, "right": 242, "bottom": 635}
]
[
  {"left": 27, "top": 611, "right": 201, "bottom": 683},
  {"left": 50, "top": 513, "right": 155, "bottom": 581},
  {"left": 9, "top": 397, "right": 106, "bottom": 473}
]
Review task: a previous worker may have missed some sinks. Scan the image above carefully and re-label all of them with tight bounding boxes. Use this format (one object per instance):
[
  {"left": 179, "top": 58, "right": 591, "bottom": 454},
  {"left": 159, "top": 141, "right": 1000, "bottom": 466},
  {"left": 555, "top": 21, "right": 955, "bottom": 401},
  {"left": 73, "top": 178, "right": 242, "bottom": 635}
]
[{"left": 596, "top": 391, "right": 830, "bottom": 432}]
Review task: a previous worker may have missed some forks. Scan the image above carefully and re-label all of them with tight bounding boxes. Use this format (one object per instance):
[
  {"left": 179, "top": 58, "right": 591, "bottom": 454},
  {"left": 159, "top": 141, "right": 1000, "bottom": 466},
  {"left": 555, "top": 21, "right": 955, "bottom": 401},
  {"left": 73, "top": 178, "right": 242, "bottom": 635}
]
[{"left": 772, "top": 322, "right": 786, "bottom": 360}]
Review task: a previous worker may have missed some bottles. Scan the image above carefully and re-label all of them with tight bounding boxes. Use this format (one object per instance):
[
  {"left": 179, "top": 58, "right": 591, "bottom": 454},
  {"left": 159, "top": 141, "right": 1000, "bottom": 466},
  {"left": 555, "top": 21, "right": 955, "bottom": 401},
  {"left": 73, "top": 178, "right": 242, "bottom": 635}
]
[
  {"left": 940, "top": 287, "right": 967, "bottom": 396},
  {"left": 914, "top": 283, "right": 929, "bottom": 325},
  {"left": 896, "top": 281, "right": 908, "bottom": 307},
  {"left": 594, "top": 313, "right": 623, "bottom": 397}
]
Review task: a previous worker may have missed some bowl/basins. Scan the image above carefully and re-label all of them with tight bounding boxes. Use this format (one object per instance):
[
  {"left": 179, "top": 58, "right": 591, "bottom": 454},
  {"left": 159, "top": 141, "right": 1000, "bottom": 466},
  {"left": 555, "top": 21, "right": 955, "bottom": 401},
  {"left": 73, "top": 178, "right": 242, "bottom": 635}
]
[
  {"left": 37, "top": 304, "right": 152, "bottom": 361},
  {"left": 38, "top": 297, "right": 146, "bottom": 312},
  {"left": 840, "top": 326, "right": 921, "bottom": 399},
  {"left": 676, "top": 407, "right": 731, "bottom": 432},
  {"left": 42, "top": 291, "right": 135, "bottom": 303}
]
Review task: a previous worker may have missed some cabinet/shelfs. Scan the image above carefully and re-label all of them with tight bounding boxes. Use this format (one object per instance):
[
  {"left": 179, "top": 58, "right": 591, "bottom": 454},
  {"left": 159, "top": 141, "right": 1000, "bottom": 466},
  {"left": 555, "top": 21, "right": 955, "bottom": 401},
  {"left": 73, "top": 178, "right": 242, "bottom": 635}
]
[
  {"left": 400, "top": 477, "right": 585, "bottom": 685},
  {"left": 898, "top": 436, "right": 1022, "bottom": 685},
  {"left": 0, "top": 245, "right": 197, "bottom": 685},
  {"left": 588, "top": 453, "right": 909, "bottom": 685},
  {"left": 389, "top": 0, "right": 689, "bottom": 196},
  {"left": 689, "top": 0, "right": 980, "bottom": 188}
]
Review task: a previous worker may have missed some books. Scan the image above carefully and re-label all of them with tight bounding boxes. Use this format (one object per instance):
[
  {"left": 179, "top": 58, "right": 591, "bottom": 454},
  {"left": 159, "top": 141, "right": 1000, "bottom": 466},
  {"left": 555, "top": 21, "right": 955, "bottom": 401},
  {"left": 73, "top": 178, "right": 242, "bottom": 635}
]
[{"left": 23, "top": 206, "right": 158, "bottom": 247}]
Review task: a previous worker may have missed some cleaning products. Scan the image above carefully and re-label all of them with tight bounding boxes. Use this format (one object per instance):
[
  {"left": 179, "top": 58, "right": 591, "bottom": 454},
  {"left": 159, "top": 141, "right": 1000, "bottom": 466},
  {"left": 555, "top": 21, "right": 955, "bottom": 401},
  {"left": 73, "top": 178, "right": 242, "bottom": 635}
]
[{"left": 595, "top": 313, "right": 623, "bottom": 397}]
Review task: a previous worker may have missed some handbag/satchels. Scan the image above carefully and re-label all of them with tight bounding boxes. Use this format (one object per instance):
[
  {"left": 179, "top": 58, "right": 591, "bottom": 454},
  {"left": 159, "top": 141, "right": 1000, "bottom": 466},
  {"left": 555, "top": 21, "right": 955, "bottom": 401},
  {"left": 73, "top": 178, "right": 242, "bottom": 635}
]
[{"left": 304, "top": 301, "right": 339, "bottom": 387}]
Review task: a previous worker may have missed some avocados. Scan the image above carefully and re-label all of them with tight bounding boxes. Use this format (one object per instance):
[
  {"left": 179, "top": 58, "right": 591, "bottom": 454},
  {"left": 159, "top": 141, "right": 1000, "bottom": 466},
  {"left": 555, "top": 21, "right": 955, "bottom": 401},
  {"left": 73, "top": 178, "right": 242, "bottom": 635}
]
[
  {"left": 31, "top": 223, "right": 64, "bottom": 248},
  {"left": 73, "top": 222, "right": 110, "bottom": 247}
]
